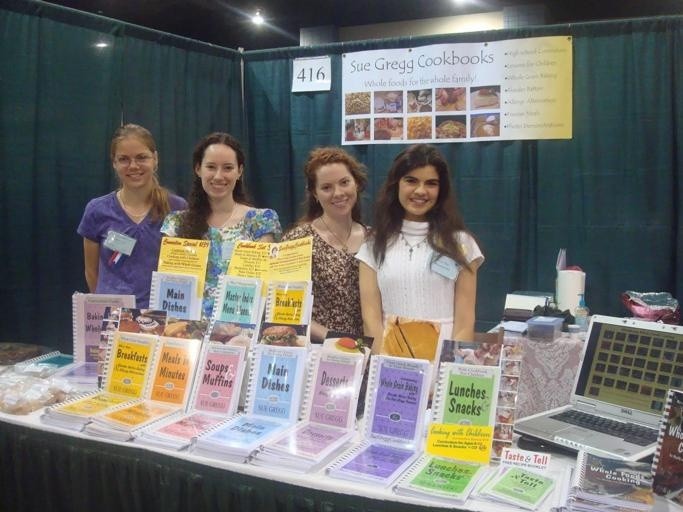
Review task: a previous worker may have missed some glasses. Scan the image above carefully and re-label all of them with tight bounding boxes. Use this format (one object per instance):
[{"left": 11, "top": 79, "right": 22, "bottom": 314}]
[{"left": 118, "top": 154, "right": 150, "bottom": 167}]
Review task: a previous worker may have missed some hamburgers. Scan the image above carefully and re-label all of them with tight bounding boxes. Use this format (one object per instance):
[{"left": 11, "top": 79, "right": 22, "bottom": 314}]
[{"left": 263, "top": 326, "right": 297, "bottom": 346}]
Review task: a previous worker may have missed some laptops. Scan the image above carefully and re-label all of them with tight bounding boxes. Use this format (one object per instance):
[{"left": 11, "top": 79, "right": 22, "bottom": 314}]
[{"left": 512, "top": 314, "right": 683, "bottom": 464}]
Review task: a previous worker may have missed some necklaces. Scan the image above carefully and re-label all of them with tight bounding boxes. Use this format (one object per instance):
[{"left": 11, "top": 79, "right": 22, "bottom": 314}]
[
  {"left": 320, "top": 215, "right": 352, "bottom": 252},
  {"left": 118, "top": 191, "right": 146, "bottom": 217},
  {"left": 399, "top": 232, "right": 428, "bottom": 260},
  {"left": 204, "top": 203, "right": 237, "bottom": 230}
]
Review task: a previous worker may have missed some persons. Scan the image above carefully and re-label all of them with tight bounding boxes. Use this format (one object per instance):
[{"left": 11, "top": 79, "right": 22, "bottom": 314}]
[
  {"left": 158, "top": 131, "right": 282, "bottom": 317},
  {"left": 282, "top": 146, "right": 373, "bottom": 340},
  {"left": 349, "top": 140, "right": 485, "bottom": 407},
  {"left": 75, "top": 122, "right": 191, "bottom": 309}
]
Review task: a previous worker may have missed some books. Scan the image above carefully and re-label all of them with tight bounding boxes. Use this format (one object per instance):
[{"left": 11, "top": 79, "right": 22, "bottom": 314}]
[
  {"left": 15, "top": 271, "right": 555, "bottom": 509},
  {"left": 650, "top": 389, "right": 682, "bottom": 508},
  {"left": 561, "top": 450, "right": 655, "bottom": 511}
]
